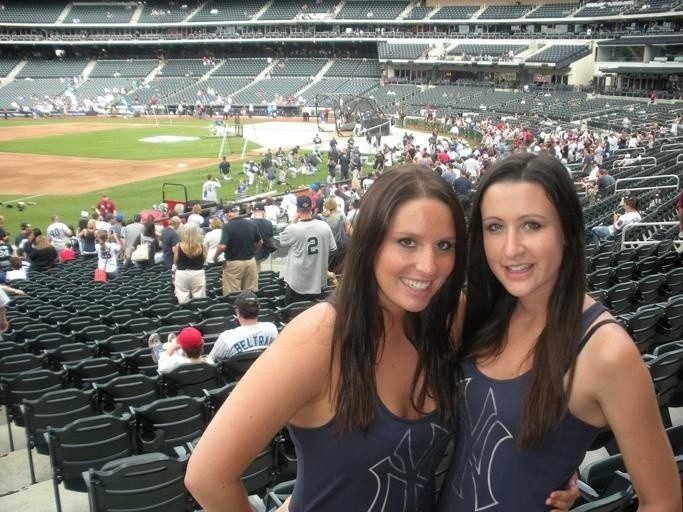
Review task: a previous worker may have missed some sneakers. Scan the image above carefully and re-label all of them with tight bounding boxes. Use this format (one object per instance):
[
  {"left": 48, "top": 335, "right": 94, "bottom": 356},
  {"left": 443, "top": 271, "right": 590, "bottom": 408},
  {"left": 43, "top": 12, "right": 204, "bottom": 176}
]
[
  {"left": 167, "top": 333, "right": 176, "bottom": 342},
  {"left": 148, "top": 333, "right": 160, "bottom": 349}
]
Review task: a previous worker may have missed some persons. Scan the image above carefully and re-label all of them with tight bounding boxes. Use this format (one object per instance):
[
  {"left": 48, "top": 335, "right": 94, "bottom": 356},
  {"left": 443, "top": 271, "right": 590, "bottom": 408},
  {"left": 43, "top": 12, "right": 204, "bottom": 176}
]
[
  {"left": 0, "top": 287, "right": 11, "bottom": 332},
  {"left": 0, "top": 284, "right": 24, "bottom": 296},
  {"left": 204, "top": 291, "right": 278, "bottom": 362},
  {"left": 170, "top": 125, "right": 683, "bottom": 304},
  {"left": 0, "top": 2, "right": 681, "bottom": 125},
  {"left": 183, "top": 160, "right": 582, "bottom": 512},
  {"left": 447, "top": 150, "right": 683, "bottom": 512},
  {"left": 0, "top": 192, "right": 171, "bottom": 283},
  {"left": 148, "top": 327, "right": 214, "bottom": 375}
]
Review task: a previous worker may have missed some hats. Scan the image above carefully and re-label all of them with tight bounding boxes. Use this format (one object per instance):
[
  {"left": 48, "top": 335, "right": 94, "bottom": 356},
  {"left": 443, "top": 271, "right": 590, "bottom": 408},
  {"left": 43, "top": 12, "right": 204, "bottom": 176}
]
[
  {"left": 296, "top": 195, "right": 311, "bottom": 208},
  {"left": 254, "top": 203, "right": 263, "bottom": 210},
  {"left": 223, "top": 204, "right": 234, "bottom": 213},
  {"left": 176, "top": 327, "right": 201, "bottom": 349},
  {"left": 234, "top": 291, "right": 258, "bottom": 307}
]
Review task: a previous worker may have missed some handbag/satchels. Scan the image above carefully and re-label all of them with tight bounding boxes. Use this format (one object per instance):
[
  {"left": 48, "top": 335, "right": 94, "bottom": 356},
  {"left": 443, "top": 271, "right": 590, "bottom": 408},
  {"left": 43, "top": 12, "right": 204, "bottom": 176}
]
[{"left": 94, "top": 269, "right": 107, "bottom": 282}]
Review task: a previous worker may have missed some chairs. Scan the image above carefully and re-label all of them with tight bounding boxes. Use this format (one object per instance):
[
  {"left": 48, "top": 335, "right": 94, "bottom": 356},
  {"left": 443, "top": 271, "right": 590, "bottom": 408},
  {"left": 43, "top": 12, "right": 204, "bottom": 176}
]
[
  {"left": 575, "top": 114, "right": 682, "bottom": 512},
  {"left": 0, "top": 252, "right": 342, "bottom": 512},
  {"left": 0, "top": 1, "right": 682, "bottom": 114},
  {"left": 414, "top": 114, "right": 573, "bottom": 144}
]
